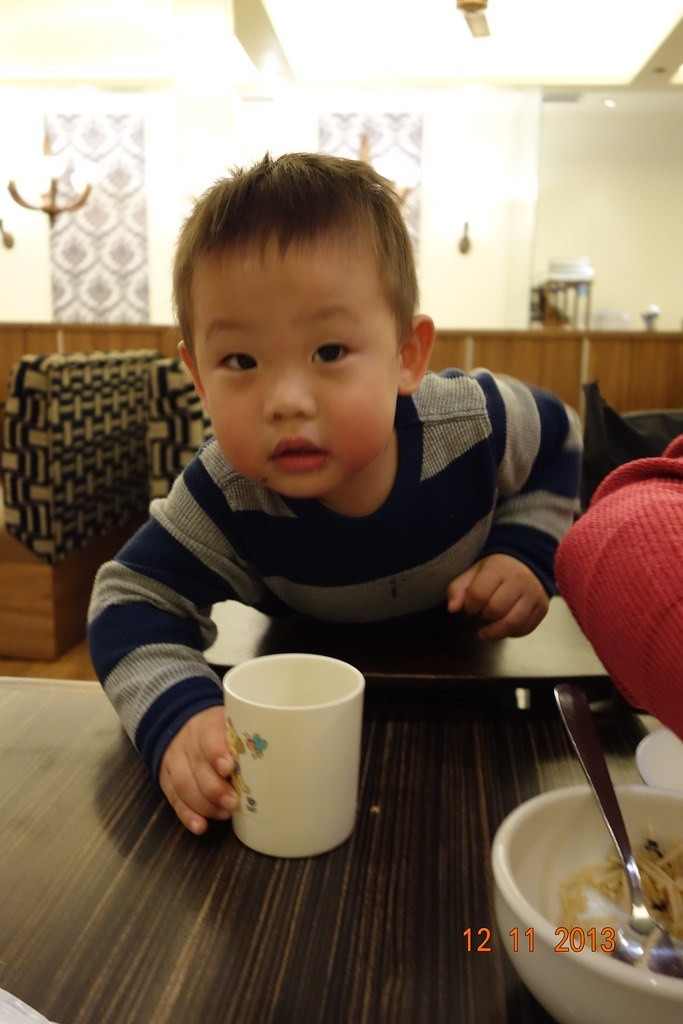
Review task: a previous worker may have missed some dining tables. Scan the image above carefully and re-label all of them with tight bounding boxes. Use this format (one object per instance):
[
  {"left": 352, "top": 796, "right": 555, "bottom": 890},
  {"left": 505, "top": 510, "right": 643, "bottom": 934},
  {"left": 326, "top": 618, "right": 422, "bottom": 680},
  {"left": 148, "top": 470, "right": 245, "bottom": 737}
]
[{"left": 0, "top": 678, "right": 682, "bottom": 1023}]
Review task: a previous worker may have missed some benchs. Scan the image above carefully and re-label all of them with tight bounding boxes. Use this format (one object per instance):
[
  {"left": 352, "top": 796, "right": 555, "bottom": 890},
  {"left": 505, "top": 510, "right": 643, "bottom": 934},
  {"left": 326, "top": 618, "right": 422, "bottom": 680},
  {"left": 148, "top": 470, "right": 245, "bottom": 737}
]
[
  {"left": 145, "top": 358, "right": 215, "bottom": 505},
  {"left": 0, "top": 346, "right": 166, "bottom": 661}
]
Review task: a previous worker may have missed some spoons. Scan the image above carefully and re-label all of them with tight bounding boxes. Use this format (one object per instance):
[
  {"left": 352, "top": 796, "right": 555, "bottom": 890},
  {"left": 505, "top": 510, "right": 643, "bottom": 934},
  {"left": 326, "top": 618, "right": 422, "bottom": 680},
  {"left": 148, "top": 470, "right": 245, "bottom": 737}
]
[{"left": 552, "top": 683, "right": 682, "bottom": 979}]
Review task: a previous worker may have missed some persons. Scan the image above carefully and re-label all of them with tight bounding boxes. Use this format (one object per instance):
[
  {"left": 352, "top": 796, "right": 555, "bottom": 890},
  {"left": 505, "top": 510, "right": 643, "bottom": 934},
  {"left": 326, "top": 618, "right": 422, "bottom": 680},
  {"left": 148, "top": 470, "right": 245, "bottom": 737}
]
[{"left": 87, "top": 150, "right": 585, "bottom": 834}]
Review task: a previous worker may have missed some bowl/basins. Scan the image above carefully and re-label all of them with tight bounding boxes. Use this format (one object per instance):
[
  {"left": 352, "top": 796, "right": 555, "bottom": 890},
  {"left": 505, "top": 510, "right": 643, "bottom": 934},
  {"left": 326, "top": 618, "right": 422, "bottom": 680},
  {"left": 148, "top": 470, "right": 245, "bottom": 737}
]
[{"left": 491, "top": 785, "right": 681, "bottom": 1022}]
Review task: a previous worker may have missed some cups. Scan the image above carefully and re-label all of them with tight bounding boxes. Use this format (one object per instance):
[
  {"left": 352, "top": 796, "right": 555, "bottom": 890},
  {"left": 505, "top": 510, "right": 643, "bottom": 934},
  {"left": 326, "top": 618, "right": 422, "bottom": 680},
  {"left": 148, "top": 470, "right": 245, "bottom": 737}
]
[{"left": 224, "top": 654, "right": 364, "bottom": 861}]
